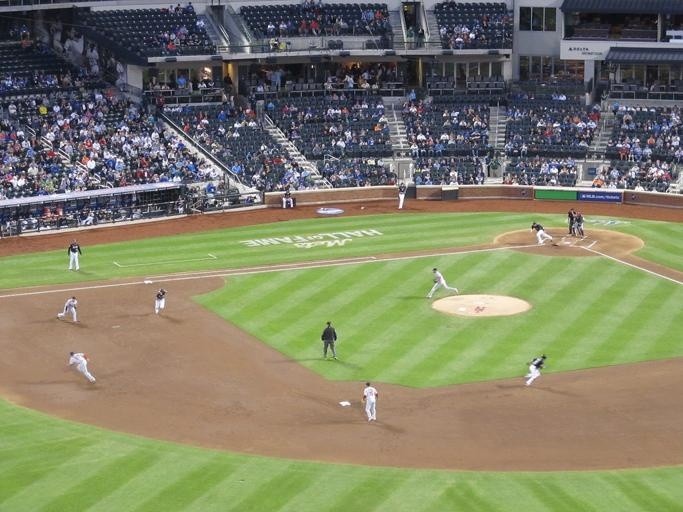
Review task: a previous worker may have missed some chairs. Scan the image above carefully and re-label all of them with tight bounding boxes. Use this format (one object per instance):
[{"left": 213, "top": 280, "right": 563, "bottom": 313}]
[{"left": 0, "top": 3, "right": 682, "bottom": 193}]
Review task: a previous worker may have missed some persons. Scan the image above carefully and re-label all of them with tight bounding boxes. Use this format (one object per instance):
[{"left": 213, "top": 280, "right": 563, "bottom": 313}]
[
  {"left": 320, "top": 321, "right": 336, "bottom": 360},
  {"left": 360, "top": 382, "right": 378, "bottom": 422},
  {"left": 66, "top": 238, "right": 81, "bottom": 272},
  {"left": 154, "top": 288, "right": 166, "bottom": 314},
  {"left": 55, "top": 295, "right": 78, "bottom": 323},
  {"left": 425, "top": 267, "right": 457, "bottom": 298},
  {"left": 523, "top": 354, "right": 546, "bottom": 386},
  {"left": 531, "top": 222, "right": 551, "bottom": 245},
  {"left": 68, "top": 351, "right": 96, "bottom": 383}
]
